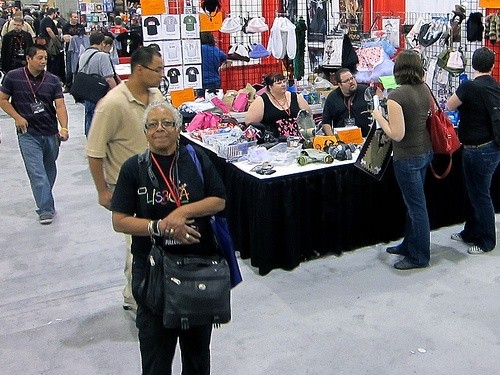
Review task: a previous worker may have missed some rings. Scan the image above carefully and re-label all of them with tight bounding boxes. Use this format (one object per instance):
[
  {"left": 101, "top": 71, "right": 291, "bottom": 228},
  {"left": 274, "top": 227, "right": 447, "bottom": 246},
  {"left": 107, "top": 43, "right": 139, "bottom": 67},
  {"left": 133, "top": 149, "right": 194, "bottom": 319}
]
[
  {"left": 185, "top": 233, "right": 190, "bottom": 239},
  {"left": 170, "top": 228, "right": 174, "bottom": 232}
]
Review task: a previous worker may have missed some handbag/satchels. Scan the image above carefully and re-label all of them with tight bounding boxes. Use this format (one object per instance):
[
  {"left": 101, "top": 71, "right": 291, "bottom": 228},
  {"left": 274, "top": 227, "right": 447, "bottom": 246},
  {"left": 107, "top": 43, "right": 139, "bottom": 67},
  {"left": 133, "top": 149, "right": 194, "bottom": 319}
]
[
  {"left": 424, "top": 82, "right": 461, "bottom": 179},
  {"left": 136, "top": 246, "right": 231, "bottom": 329},
  {"left": 232, "top": 93, "right": 249, "bottom": 111},
  {"left": 186, "top": 144, "right": 243, "bottom": 290},
  {"left": 70, "top": 72, "right": 110, "bottom": 104}
]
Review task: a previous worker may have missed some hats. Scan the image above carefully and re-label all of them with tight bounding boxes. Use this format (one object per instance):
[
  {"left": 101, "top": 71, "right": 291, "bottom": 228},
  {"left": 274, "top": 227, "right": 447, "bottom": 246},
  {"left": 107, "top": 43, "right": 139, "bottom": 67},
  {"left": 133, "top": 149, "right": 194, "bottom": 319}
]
[
  {"left": 219, "top": 18, "right": 240, "bottom": 34},
  {"left": 228, "top": 45, "right": 250, "bottom": 62},
  {"left": 245, "top": 18, "right": 268, "bottom": 32},
  {"left": 249, "top": 44, "right": 270, "bottom": 59}
]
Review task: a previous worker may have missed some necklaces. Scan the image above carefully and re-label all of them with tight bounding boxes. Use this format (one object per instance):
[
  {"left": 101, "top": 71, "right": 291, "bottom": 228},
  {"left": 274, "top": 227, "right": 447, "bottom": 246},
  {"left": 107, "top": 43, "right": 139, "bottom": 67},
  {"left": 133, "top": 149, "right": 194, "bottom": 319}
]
[
  {"left": 269, "top": 90, "right": 290, "bottom": 116},
  {"left": 151, "top": 152, "right": 181, "bottom": 207}
]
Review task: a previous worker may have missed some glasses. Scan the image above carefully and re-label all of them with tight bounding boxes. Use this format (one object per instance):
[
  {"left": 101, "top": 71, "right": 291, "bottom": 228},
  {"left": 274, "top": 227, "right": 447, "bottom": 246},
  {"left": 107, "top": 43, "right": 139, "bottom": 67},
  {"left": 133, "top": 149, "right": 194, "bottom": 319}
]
[
  {"left": 140, "top": 64, "right": 164, "bottom": 73},
  {"left": 344, "top": 75, "right": 356, "bottom": 84},
  {"left": 145, "top": 121, "right": 175, "bottom": 129}
]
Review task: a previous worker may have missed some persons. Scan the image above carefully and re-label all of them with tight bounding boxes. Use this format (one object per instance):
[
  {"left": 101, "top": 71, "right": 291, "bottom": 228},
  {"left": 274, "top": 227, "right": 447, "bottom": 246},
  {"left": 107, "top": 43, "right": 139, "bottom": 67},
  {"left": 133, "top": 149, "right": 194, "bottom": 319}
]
[
  {"left": 0, "top": 0, "right": 143, "bottom": 224},
  {"left": 112, "top": 100, "right": 241, "bottom": 375},
  {"left": 446, "top": 47, "right": 500, "bottom": 254},
  {"left": 198, "top": 31, "right": 227, "bottom": 98},
  {"left": 320, "top": 68, "right": 382, "bottom": 138},
  {"left": 245, "top": 73, "right": 314, "bottom": 139},
  {"left": 85, "top": 46, "right": 166, "bottom": 322},
  {"left": 370, "top": 49, "right": 437, "bottom": 269}
]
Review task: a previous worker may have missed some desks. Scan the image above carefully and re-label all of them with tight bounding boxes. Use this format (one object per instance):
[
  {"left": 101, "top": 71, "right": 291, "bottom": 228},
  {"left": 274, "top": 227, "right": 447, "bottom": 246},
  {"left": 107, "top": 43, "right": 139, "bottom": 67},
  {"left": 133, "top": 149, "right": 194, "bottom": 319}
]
[
  {"left": 179, "top": 123, "right": 500, "bottom": 275},
  {"left": 179, "top": 98, "right": 323, "bottom": 123}
]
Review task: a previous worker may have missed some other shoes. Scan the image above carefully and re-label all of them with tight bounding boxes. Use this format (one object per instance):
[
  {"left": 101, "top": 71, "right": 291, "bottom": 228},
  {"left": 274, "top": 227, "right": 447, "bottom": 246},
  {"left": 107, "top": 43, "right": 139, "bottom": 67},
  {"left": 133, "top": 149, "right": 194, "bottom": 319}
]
[
  {"left": 468, "top": 245, "right": 484, "bottom": 254},
  {"left": 40, "top": 214, "right": 52, "bottom": 224},
  {"left": 123, "top": 305, "right": 137, "bottom": 320},
  {"left": 394, "top": 259, "right": 416, "bottom": 270},
  {"left": 451, "top": 233, "right": 464, "bottom": 241},
  {"left": 386, "top": 247, "right": 398, "bottom": 254}
]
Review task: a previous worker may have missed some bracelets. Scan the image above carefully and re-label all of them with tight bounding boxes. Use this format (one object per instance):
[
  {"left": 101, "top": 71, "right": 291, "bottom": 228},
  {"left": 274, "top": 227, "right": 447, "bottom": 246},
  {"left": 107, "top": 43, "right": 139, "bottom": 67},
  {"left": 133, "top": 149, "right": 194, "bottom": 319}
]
[
  {"left": 148, "top": 218, "right": 161, "bottom": 237},
  {"left": 60, "top": 128, "right": 68, "bottom": 132}
]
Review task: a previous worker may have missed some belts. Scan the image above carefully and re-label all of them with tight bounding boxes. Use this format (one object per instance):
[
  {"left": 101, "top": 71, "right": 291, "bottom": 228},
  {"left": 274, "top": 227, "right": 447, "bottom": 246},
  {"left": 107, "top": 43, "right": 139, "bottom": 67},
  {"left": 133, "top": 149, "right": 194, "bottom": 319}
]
[{"left": 464, "top": 140, "right": 494, "bottom": 149}]
